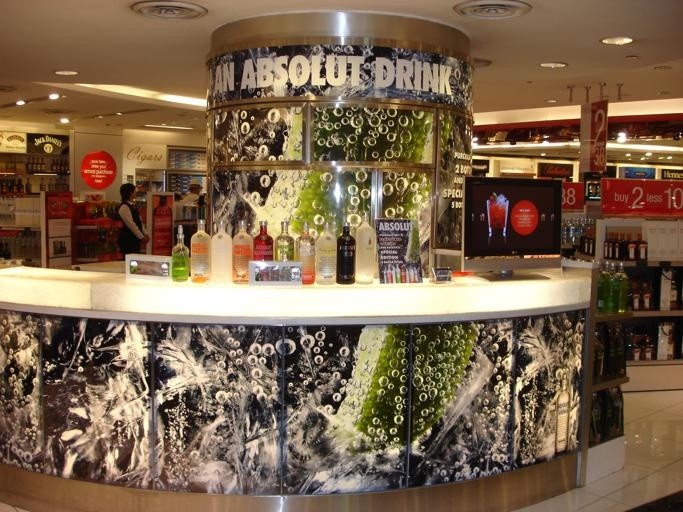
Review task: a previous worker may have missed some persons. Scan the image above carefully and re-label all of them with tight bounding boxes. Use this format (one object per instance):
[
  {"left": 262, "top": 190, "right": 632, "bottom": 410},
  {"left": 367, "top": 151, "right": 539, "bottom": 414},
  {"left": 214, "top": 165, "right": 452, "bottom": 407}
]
[
  {"left": 117, "top": 183, "right": 149, "bottom": 260},
  {"left": 181, "top": 179, "right": 203, "bottom": 203}
]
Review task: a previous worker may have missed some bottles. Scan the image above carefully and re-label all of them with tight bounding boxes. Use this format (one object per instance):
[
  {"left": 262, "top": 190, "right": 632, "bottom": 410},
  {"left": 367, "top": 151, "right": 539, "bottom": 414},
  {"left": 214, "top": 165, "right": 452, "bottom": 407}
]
[
  {"left": 25, "top": 157, "right": 32, "bottom": 173},
  {"left": 337, "top": 221, "right": 355, "bottom": 284},
  {"left": 313, "top": 221, "right": 337, "bottom": 286},
  {"left": 355, "top": 216, "right": 376, "bottom": 285},
  {"left": 189, "top": 218, "right": 209, "bottom": 283},
  {"left": 293, "top": 220, "right": 314, "bottom": 283},
  {"left": 252, "top": 220, "right": 273, "bottom": 262},
  {"left": 76, "top": 228, "right": 120, "bottom": 259},
  {"left": 208, "top": 219, "right": 232, "bottom": 284},
  {"left": 589, "top": 384, "right": 624, "bottom": 447},
  {"left": 50, "top": 157, "right": 68, "bottom": 172},
  {"left": 31, "top": 156, "right": 36, "bottom": 173},
  {"left": 39, "top": 177, "right": 68, "bottom": 192},
  {"left": 560, "top": 215, "right": 596, "bottom": 258},
  {"left": 0, "top": 174, "right": 30, "bottom": 195},
  {"left": 602, "top": 232, "right": 647, "bottom": 261},
  {"left": 562, "top": 255, "right": 652, "bottom": 315},
  {"left": 485, "top": 200, "right": 509, "bottom": 240},
  {"left": 152, "top": 195, "right": 172, "bottom": 256},
  {"left": 170, "top": 224, "right": 189, "bottom": 282},
  {"left": 230, "top": 219, "right": 253, "bottom": 284},
  {"left": 87, "top": 201, "right": 122, "bottom": 221},
  {"left": 378, "top": 262, "right": 421, "bottom": 284},
  {"left": 0, "top": 231, "right": 40, "bottom": 260},
  {"left": 670, "top": 270, "right": 678, "bottom": 310},
  {"left": 591, "top": 319, "right": 655, "bottom": 381},
  {"left": 274, "top": 221, "right": 294, "bottom": 263},
  {"left": 0, "top": 154, "right": 16, "bottom": 173},
  {"left": 40, "top": 157, "right": 45, "bottom": 173},
  {"left": 35, "top": 157, "right": 40, "bottom": 173}
]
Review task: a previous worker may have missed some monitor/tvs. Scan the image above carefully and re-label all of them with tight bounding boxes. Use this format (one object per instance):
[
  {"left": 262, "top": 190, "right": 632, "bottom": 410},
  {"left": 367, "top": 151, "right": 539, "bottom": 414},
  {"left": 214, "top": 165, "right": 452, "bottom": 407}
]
[{"left": 462, "top": 176, "right": 562, "bottom": 277}]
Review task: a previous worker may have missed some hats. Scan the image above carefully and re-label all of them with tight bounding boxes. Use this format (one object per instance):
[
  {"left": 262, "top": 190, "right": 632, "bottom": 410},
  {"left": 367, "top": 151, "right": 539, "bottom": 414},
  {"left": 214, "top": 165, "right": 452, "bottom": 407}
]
[{"left": 187, "top": 179, "right": 203, "bottom": 188}]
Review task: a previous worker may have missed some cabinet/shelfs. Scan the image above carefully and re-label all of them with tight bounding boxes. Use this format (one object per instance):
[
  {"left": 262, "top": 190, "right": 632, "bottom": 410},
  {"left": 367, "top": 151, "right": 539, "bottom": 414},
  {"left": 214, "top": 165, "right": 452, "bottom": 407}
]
[
  {"left": 586, "top": 260, "right": 631, "bottom": 450},
  {"left": 571, "top": 248, "right": 682, "bottom": 366},
  {"left": 0, "top": 166, "right": 209, "bottom": 270}
]
